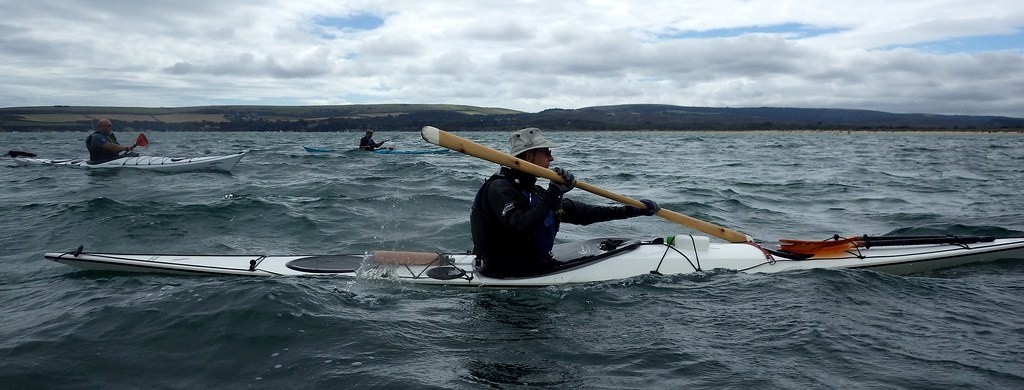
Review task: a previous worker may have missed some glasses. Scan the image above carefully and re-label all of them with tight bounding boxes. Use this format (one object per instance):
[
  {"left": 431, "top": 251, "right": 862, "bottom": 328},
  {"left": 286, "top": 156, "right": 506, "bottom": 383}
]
[
  {"left": 104, "top": 124, "right": 112, "bottom": 127},
  {"left": 532, "top": 148, "right": 551, "bottom": 157}
]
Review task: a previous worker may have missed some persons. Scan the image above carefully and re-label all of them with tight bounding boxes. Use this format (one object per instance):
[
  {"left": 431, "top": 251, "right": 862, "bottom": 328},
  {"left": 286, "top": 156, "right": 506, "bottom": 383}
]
[
  {"left": 89, "top": 119, "right": 133, "bottom": 163},
  {"left": 470, "top": 128, "right": 660, "bottom": 280},
  {"left": 359, "top": 129, "right": 383, "bottom": 151}
]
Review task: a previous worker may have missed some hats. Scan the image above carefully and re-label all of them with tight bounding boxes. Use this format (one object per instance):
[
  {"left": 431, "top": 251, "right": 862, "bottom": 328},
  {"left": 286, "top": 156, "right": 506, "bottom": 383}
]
[
  {"left": 366, "top": 129, "right": 374, "bottom": 134},
  {"left": 508, "top": 127, "right": 561, "bottom": 157}
]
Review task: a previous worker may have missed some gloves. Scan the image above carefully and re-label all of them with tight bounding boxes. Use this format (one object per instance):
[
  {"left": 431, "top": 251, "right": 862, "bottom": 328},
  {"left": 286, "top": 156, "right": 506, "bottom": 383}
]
[
  {"left": 625, "top": 199, "right": 660, "bottom": 217},
  {"left": 550, "top": 167, "right": 577, "bottom": 194}
]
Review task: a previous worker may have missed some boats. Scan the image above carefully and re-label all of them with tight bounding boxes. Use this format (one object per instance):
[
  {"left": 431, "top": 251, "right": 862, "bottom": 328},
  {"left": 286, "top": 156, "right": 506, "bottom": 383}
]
[
  {"left": 44, "top": 224, "right": 1024, "bottom": 291},
  {"left": 303, "top": 145, "right": 450, "bottom": 154},
  {"left": 10, "top": 151, "right": 246, "bottom": 172}
]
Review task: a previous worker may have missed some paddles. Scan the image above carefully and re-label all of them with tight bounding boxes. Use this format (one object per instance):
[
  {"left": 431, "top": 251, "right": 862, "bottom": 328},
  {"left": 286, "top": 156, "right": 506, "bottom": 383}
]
[
  {"left": 421, "top": 124, "right": 815, "bottom": 260},
  {"left": 365, "top": 134, "right": 400, "bottom": 150},
  {"left": 120, "top": 133, "right": 149, "bottom": 157},
  {"left": 778, "top": 233, "right": 996, "bottom": 258}
]
